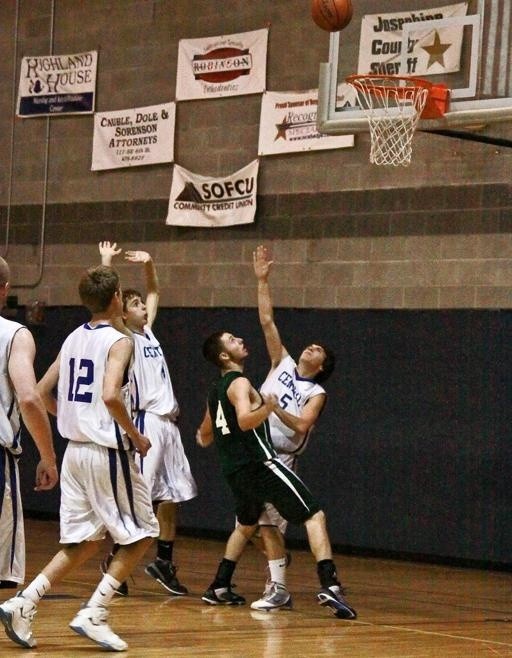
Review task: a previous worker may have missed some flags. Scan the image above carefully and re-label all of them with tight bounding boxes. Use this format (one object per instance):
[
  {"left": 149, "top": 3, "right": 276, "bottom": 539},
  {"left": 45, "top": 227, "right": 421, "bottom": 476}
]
[
  {"left": 15, "top": 48, "right": 99, "bottom": 118},
  {"left": 354, "top": 0, "right": 469, "bottom": 82},
  {"left": 257, "top": 80, "right": 356, "bottom": 156},
  {"left": 163, "top": 155, "right": 260, "bottom": 227},
  {"left": 90, "top": 100, "right": 177, "bottom": 172},
  {"left": 175, "top": 25, "right": 271, "bottom": 104}
]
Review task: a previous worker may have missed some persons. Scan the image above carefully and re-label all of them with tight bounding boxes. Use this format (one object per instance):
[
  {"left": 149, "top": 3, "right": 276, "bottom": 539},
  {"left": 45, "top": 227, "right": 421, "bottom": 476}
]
[
  {"left": 1, "top": 258, "right": 60, "bottom": 593},
  {"left": 229, "top": 243, "right": 335, "bottom": 614},
  {"left": 2, "top": 263, "right": 161, "bottom": 652},
  {"left": 194, "top": 328, "right": 359, "bottom": 620},
  {"left": 97, "top": 239, "right": 202, "bottom": 597}
]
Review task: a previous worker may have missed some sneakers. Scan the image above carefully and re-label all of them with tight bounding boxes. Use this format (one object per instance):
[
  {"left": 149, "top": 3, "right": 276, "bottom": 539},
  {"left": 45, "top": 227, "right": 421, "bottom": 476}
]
[
  {"left": 314, "top": 586, "right": 356, "bottom": 620},
  {"left": 2, "top": 590, "right": 37, "bottom": 647},
  {"left": 69, "top": 604, "right": 127, "bottom": 650},
  {"left": 202, "top": 584, "right": 245, "bottom": 605},
  {"left": 251, "top": 582, "right": 294, "bottom": 612},
  {"left": 100, "top": 552, "right": 129, "bottom": 596},
  {"left": 146, "top": 558, "right": 187, "bottom": 594}
]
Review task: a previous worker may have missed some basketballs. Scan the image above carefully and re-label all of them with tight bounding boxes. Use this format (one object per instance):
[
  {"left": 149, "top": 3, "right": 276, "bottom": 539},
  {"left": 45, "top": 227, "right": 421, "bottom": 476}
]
[{"left": 313, "top": 0, "right": 352, "bottom": 32}]
